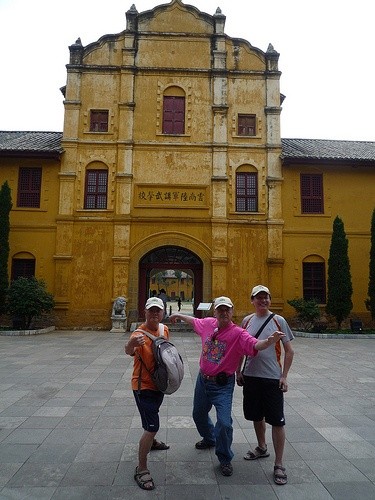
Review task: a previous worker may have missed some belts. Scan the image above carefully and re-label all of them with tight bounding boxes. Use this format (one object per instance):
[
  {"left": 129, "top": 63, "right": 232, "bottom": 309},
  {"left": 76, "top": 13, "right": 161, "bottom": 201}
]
[{"left": 199, "top": 371, "right": 217, "bottom": 381}]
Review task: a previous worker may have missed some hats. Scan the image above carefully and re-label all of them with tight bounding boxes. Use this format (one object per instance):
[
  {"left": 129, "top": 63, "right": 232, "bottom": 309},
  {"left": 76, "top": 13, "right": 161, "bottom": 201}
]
[
  {"left": 214, "top": 297, "right": 234, "bottom": 310},
  {"left": 250, "top": 285, "right": 271, "bottom": 300},
  {"left": 145, "top": 297, "right": 164, "bottom": 309}
]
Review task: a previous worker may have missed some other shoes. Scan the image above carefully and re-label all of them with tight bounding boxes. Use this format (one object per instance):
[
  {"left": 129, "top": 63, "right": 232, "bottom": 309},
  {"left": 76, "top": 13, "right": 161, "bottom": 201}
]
[
  {"left": 195, "top": 440, "right": 214, "bottom": 449},
  {"left": 219, "top": 463, "right": 234, "bottom": 476}
]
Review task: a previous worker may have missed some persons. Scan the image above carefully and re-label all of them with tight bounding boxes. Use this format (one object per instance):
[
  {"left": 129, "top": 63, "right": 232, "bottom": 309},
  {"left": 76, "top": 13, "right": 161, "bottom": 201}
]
[
  {"left": 125, "top": 297, "right": 169, "bottom": 491},
  {"left": 236, "top": 285, "right": 295, "bottom": 485},
  {"left": 168, "top": 297, "right": 286, "bottom": 477},
  {"left": 152, "top": 289, "right": 167, "bottom": 312},
  {"left": 177, "top": 298, "right": 181, "bottom": 311}
]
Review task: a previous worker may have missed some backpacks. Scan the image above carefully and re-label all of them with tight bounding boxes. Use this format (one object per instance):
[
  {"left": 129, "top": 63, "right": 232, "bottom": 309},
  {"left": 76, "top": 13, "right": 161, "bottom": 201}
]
[{"left": 131, "top": 323, "right": 184, "bottom": 395}]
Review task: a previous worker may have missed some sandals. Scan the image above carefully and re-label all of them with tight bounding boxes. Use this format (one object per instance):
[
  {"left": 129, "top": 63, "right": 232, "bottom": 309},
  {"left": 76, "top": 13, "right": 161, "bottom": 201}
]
[
  {"left": 244, "top": 444, "right": 270, "bottom": 460},
  {"left": 274, "top": 466, "right": 287, "bottom": 484},
  {"left": 134, "top": 466, "right": 155, "bottom": 490},
  {"left": 150, "top": 439, "right": 169, "bottom": 450}
]
